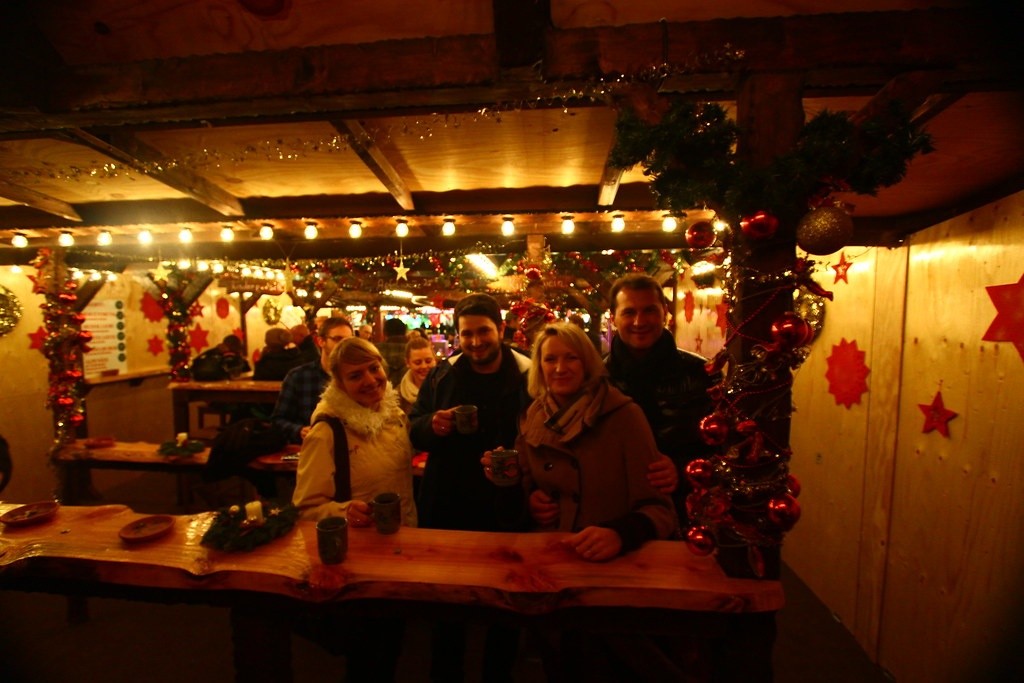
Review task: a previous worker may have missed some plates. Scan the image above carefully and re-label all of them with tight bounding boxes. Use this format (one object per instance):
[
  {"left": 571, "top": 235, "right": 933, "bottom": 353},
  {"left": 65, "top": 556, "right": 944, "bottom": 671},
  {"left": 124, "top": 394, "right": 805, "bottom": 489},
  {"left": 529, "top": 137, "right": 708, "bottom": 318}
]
[
  {"left": 117, "top": 513, "right": 178, "bottom": 545},
  {"left": 0, "top": 500, "right": 60, "bottom": 527},
  {"left": 282, "top": 452, "right": 306, "bottom": 460}
]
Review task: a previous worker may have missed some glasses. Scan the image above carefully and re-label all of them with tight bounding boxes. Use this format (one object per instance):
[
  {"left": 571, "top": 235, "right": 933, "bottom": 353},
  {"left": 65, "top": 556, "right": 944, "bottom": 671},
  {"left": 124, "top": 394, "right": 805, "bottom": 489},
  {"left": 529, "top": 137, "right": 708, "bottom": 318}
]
[{"left": 328, "top": 334, "right": 342, "bottom": 342}]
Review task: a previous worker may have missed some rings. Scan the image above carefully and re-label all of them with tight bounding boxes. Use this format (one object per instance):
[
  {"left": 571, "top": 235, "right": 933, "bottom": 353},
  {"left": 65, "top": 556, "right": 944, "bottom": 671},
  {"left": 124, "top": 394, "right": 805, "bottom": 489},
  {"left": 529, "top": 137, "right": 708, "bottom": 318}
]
[
  {"left": 588, "top": 547, "right": 595, "bottom": 555},
  {"left": 442, "top": 427, "right": 444, "bottom": 430},
  {"left": 356, "top": 519, "right": 359, "bottom": 522}
]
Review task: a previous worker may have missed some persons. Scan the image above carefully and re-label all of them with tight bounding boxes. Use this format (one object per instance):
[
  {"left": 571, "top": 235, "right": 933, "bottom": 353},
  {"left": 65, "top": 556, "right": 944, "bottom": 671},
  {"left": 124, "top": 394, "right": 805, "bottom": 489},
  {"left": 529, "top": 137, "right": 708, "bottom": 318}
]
[
  {"left": 288, "top": 336, "right": 417, "bottom": 530},
  {"left": 526, "top": 274, "right": 726, "bottom": 542},
  {"left": 407, "top": 294, "right": 537, "bottom": 534},
  {"left": 479, "top": 321, "right": 678, "bottom": 564},
  {"left": 190, "top": 319, "right": 464, "bottom": 420}
]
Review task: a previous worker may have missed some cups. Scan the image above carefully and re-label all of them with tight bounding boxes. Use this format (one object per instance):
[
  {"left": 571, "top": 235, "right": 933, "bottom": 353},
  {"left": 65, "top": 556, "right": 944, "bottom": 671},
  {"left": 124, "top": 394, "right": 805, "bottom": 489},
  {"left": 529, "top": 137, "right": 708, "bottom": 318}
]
[
  {"left": 489, "top": 449, "right": 521, "bottom": 487},
  {"left": 454, "top": 405, "right": 480, "bottom": 433},
  {"left": 317, "top": 515, "right": 348, "bottom": 566},
  {"left": 367, "top": 491, "right": 401, "bottom": 535}
]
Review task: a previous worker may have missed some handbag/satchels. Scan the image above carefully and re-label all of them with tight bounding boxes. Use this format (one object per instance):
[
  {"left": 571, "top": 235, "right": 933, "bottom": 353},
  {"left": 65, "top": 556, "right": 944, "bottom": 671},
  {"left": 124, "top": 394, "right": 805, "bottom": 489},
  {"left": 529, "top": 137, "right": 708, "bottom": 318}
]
[
  {"left": 293, "top": 603, "right": 365, "bottom": 655},
  {"left": 216, "top": 416, "right": 286, "bottom": 459}
]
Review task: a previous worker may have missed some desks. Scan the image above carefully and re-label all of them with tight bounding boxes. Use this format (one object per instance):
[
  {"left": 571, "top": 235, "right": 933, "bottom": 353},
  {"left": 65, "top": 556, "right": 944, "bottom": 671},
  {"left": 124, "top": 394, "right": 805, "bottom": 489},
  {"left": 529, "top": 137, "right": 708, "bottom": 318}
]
[
  {"left": 168, "top": 381, "right": 281, "bottom": 436},
  {"left": 47, "top": 439, "right": 425, "bottom": 506},
  {"left": 0, "top": 500, "right": 784, "bottom": 683}
]
